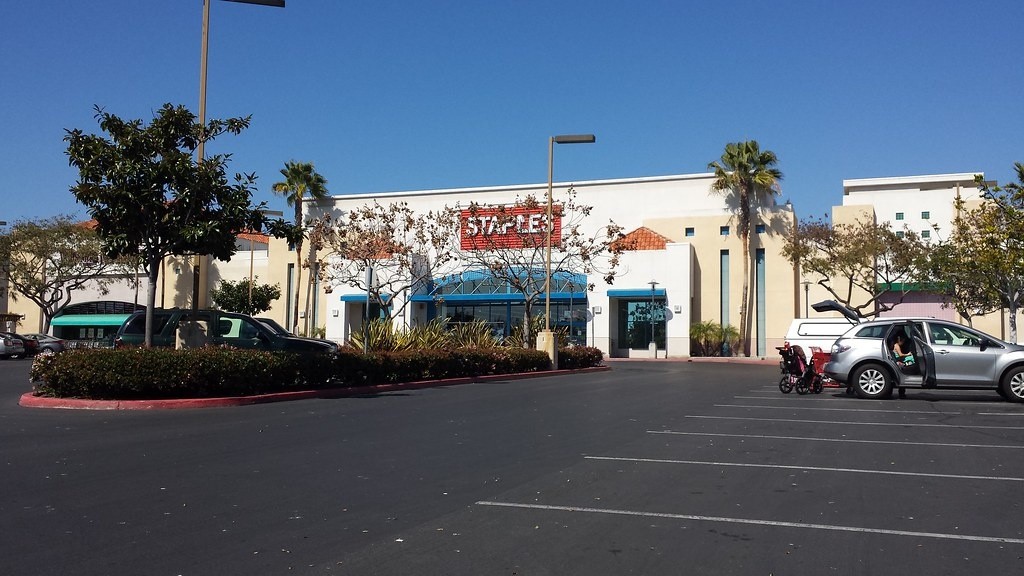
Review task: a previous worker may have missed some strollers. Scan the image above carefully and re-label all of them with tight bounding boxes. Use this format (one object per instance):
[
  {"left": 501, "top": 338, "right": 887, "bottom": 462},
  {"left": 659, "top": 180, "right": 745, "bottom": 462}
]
[{"left": 775, "top": 342, "right": 825, "bottom": 394}]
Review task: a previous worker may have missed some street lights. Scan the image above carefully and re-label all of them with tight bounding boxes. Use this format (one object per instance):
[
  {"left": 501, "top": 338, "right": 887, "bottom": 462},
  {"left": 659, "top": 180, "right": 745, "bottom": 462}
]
[
  {"left": 542, "top": 132, "right": 596, "bottom": 334},
  {"left": 196, "top": 0, "right": 287, "bottom": 178},
  {"left": 648, "top": 279, "right": 660, "bottom": 342}
]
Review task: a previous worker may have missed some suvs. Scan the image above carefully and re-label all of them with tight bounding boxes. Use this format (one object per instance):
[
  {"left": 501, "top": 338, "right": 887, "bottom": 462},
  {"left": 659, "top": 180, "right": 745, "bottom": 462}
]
[{"left": 113, "top": 306, "right": 334, "bottom": 356}]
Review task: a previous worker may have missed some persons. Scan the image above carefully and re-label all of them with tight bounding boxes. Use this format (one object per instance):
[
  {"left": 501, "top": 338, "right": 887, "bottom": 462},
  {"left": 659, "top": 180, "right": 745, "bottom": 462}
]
[{"left": 893, "top": 335, "right": 914, "bottom": 366}]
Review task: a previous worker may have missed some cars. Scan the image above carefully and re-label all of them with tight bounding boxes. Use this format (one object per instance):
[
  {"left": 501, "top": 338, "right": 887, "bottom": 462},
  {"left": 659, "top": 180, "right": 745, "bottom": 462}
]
[
  {"left": 216, "top": 317, "right": 340, "bottom": 354},
  {"left": 0, "top": 331, "right": 39, "bottom": 359},
  {"left": 0, "top": 333, "right": 25, "bottom": 360},
  {"left": 820, "top": 316, "right": 1024, "bottom": 402},
  {"left": 18, "top": 333, "right": 71, "bottom": 355}
]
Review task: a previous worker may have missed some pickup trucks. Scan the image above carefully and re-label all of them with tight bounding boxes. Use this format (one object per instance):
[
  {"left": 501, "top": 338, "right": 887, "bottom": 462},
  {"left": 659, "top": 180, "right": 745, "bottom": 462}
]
[{"left": 783, "top": 317, "right": 937, "bottom": 370}]
